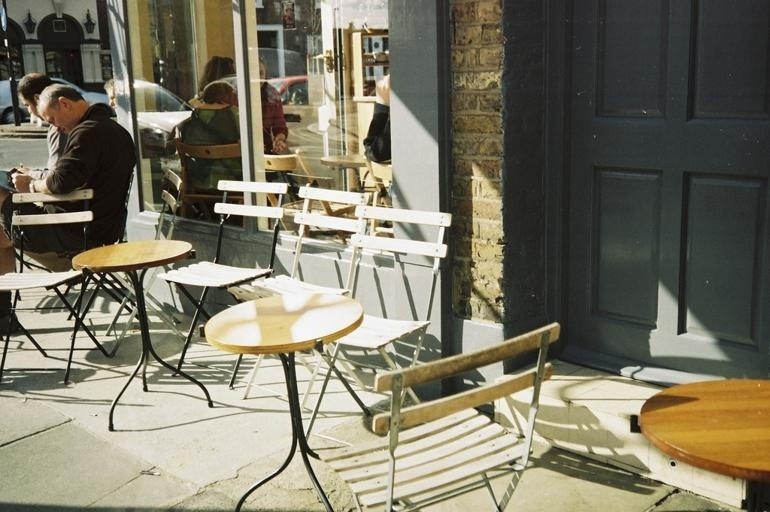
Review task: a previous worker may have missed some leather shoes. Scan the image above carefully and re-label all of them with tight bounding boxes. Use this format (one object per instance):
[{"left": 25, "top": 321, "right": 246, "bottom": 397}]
[{"left": 0, "top": 310, "right": 19, "bottom": 335}]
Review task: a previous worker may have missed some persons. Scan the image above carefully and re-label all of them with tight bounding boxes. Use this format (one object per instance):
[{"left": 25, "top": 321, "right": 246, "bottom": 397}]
[
  {"left": 198, "top": 55, "right": 236, "bottom": 95},
  {"left": 234, "top": 56, "right": 289, "bottom": 181},
  {"left": 11, "top": 71, "right": 72, "bottom": 178},
  {"left": 0, "top": 169, "right": 26, "bottom": 337},
  {"left": 179, "top": 79, "right": 251, "bottom": 226},
  {"left": 361, "top": 72, "right": 391, "bottom": 167},
  {"left": 1, "top": 79, "right": 138, "bottom": 256}
]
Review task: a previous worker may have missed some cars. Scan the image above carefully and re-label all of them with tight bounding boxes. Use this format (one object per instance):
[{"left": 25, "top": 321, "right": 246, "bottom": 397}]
[
  {"left": 0, "top": 78, "right": 116, "bottom": 125},
  {"left": 116, "top": 47, "right": 316, "bottom": 162}
]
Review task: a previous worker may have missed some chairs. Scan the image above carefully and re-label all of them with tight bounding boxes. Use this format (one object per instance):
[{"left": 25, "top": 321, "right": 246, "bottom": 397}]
[
  {"left": 314, "top": 205, "right": 455, "bottom": 429},
  {"left": 45, "top": 167, "right": 135, "bottom": 324},
  {"left": 169, "top": 139, "right": 392, "bottom": 258},
  {"left": 0, "top": 181, "right": 109, "bottom": 386},
  {"left": 171, "top": 178, "right": 292, "bottom": 390},
  {"left": 240, "top": 181, "right": 374, "bottom": 411},
  {"left": 318, "top": 319, "right": 563, "bottom": 511},
  {"left": 102, "top": 167, "right": 189, "bottom": 357}
]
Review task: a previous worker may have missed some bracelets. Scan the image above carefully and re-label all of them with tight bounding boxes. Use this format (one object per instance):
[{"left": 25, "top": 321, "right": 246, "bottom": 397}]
[{"left": 28, "top": 180, "right": 36, "bottom": 194}]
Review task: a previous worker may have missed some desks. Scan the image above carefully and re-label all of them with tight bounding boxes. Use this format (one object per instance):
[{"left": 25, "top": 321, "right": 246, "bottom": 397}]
[
  {"left": 65, "top": 238, "right": 213, "bottom": 432},
  {"left": 202, "top": 292, "right": 365, "bottom": 512},
  {"left": 640, "top": 377, "right": 769, "bottom": 512}
]
